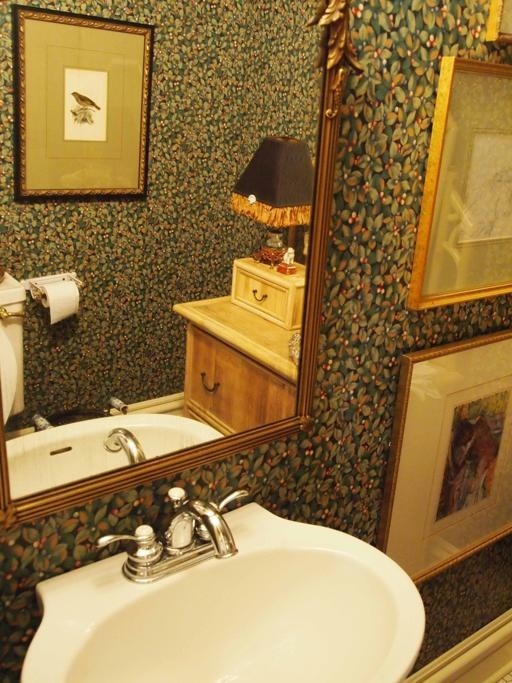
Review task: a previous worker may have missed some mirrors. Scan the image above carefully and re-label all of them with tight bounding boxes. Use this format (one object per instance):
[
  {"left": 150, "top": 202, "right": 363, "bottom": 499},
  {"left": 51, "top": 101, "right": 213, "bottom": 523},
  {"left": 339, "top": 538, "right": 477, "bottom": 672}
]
[{"left": 0, "top": 0, "right": 348, "bottom": 530}]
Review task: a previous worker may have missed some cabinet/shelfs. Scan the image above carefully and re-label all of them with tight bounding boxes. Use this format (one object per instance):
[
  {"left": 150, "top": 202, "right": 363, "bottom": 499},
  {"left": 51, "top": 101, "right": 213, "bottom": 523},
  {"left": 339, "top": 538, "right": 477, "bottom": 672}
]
[{"left": 177, "top": 317, "right": 297, "bottom": 435}]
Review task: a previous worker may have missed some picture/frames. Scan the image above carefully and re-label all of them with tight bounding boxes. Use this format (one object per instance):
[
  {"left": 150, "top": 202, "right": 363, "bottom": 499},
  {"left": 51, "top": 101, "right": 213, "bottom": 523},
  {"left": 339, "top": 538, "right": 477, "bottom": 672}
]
[
  {"left": 13, "top": 3, "right": 151, "bottom": 201},
  {"left": 404, "top": 57, "right": 512, "bottom": 310},
  {"left": 380, "top": 328, "right": 512, "bottom": 589},
  {"left": 483, "top": 0, "right": 510, "bottom": 47}
]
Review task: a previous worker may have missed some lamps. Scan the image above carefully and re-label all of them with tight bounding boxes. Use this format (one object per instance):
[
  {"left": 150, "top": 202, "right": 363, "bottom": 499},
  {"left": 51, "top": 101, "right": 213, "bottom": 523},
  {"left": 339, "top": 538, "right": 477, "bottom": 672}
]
[{"left": 222, "top": 134, "right": 317, "bottom": 266}]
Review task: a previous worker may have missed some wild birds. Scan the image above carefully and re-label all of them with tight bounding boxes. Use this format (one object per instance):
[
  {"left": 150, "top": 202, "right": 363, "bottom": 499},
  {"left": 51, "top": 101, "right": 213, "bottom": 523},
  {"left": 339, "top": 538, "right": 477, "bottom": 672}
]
[{"left": 70, "top": 91, "right": 101, "bottom": 111}]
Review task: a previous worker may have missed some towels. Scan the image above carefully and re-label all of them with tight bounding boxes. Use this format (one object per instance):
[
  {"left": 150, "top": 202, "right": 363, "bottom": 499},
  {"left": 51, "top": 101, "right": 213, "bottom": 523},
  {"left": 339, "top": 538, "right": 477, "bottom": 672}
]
[{"left": 0, "top": 305, "right": 28, "bottom": 425}]
